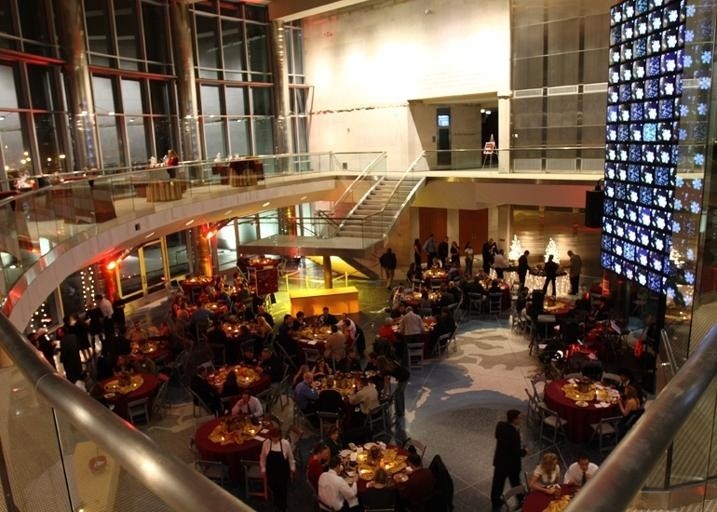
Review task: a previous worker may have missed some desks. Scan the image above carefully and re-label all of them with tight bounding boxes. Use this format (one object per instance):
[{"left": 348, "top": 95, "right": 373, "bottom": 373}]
[{"left": 3, "top": 156, "right": 266, "bottom": 235}]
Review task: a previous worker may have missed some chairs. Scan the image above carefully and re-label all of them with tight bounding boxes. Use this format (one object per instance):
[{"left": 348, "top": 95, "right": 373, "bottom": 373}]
[{"left": 95, "top": 253, "right": 645, "bottom": 510}]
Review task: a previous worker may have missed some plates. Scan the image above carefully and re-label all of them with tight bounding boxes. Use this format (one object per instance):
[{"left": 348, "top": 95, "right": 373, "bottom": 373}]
[
  {"left": 560, "top": 377, "right": 619, "bottom": 408},
  {"left": 546, "top": 483, "right": 561, "bottom": 489},
  {"left": 364, "top": 369, "right": 376, "bottom": 377},
  {"left": 334, "top": 441, "right": 412, "bottom": 489}
]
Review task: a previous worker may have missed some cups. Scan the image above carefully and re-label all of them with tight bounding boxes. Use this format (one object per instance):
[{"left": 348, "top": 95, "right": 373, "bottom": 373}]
[
  {"left": 149, "top": 155, "right": 167, "bottom": 167},
  {"left": 215, "top": 152, "right": 240, "bottom": 161}
]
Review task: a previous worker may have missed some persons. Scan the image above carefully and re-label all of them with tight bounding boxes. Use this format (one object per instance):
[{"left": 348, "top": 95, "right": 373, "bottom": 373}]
[
  {"left": 413, "top": 234, "right": 473, "bottom": 362},
  {"left": 557, "top": 292, "right": 657, "bottom": 432},
  {"left": 542, "top": 250, "right": 582, "bottom": 297},
  {"left": 37, "top": 254, "right": 436, "bottom": 512},
  {"left": 379, "top": 248, "right": 397, "bottom": 291},
  {"left": 490, "top": 409, "right": 600, "bottom": 509},
  {"left": 468, "top": 279, "right": 550, "bottom": 340},
  {"left": 164, "top": 148, "right": 180, "bottom": 176},
  {"left": 482, "top": 239, "right": 531, "bottom": 287}
]
